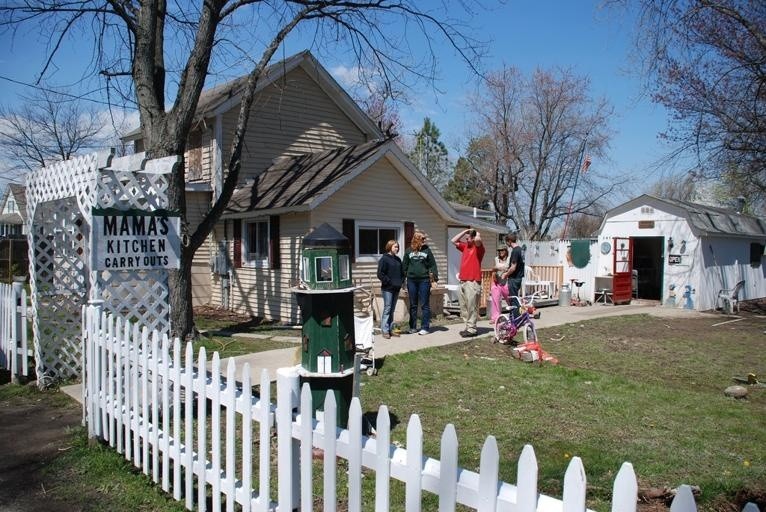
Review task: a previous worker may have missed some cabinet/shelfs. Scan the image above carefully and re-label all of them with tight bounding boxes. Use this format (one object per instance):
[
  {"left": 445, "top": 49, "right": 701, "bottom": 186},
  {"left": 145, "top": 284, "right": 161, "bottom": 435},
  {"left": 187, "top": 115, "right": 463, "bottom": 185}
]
[
  {"left": 525, "top": 278, "right": 556, "bottom": 300},
  {"left": 441, "top": 284, "right": 468, "bottom": 309}
]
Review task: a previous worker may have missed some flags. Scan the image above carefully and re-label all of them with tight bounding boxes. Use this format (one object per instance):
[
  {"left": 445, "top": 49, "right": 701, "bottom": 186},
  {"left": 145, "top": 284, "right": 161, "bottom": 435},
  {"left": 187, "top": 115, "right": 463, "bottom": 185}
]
[{"left": 582, "top": 139, "right": 592, "bottom": 175}]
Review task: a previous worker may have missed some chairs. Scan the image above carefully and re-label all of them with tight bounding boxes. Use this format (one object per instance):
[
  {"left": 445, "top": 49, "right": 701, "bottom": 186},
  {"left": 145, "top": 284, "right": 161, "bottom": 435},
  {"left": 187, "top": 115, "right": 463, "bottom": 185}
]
[{"left": 712, "top": 278, "right": 747, "bottom": 315}]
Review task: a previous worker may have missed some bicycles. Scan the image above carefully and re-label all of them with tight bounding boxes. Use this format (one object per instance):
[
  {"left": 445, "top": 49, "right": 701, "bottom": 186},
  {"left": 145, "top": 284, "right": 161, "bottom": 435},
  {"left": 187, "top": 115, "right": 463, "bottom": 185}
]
[{"left": 494, "top": 291, "right": 546, "bottom": 347}]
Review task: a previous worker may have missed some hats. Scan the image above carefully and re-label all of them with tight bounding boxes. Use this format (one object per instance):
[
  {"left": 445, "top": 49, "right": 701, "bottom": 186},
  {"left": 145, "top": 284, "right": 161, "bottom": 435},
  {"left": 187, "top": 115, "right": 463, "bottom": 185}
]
[{"left": 496, "top": 244, "right": 506, "bottom": 250}]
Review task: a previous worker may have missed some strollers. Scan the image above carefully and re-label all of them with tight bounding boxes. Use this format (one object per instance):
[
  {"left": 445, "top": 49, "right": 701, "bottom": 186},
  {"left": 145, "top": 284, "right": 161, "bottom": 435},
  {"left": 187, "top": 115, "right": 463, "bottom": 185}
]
[{"left": 353, "top": 309, "right": 377, "bottom": 377}]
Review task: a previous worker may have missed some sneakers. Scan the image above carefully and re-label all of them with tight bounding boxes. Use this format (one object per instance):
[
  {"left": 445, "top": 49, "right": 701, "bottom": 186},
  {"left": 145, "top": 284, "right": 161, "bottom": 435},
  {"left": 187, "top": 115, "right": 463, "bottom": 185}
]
[
  {"left": 408, "top": 329, "right": 417, "bottom": 333},
  {"left": 418, "top": 330, "right": 428, "bottom": 335},
  {"left": 460, "top": 330, "right": 477, "bottom": 337},
  {"left": 383, "top": 334, "right": 390, "bottom": 338},
  {"left": 390, "top": 332, "right": 399, "bottom": 336}
]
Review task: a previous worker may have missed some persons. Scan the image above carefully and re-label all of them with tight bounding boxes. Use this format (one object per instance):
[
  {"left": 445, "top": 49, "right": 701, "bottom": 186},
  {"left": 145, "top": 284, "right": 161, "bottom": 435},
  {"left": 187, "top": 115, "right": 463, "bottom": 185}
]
[
  {"left": 377, "top": 239, "right": 404, "bottom": 340},
  {"left": 490, "top": 243, "right": 510, "bottom": 325},
  {"left": 501, "top": 233, "right": 526, "bottom": 326},
  {"left": 450, "top": 229, "right": 486, "bottom": 338},
  {"left": 403, "top": 231, "right": 439, "bottom": 335}
]
